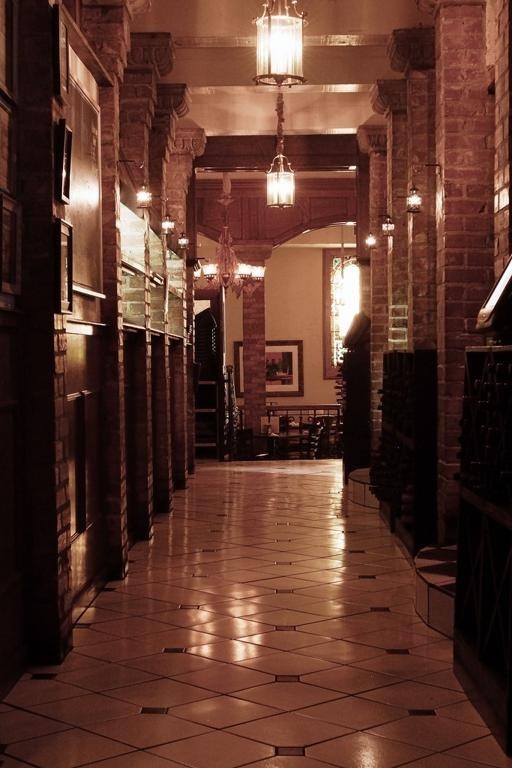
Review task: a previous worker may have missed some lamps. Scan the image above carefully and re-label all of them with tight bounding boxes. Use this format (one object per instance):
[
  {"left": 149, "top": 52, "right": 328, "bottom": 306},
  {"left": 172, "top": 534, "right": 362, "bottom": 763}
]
[
  {"left": 376, "top": 163, "right": 443, "bottom": 236},
  {"left": 118, "top": 158, "right": 189, "bottom": 248},
  {"left": 193, "top": 171, "right": 266, "bottom": 298},
  {"left": 248, "top": 1, "right": 309, "bottom": 211}
]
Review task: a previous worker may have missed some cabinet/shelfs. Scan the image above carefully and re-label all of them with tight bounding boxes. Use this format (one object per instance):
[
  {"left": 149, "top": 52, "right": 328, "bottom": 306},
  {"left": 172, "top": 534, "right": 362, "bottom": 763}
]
[{"left": 194, "top": 379, "right": 224, "bottom": 458}]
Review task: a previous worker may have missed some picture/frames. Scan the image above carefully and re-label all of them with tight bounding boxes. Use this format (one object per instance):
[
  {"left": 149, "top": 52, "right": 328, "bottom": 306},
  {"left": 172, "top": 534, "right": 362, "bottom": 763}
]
[
  {"left": 232, "top": 337, "right": 305, "bottom": 397},
  {"left": 54, "top": 116, "right": 73, "bottom": 316}
]
